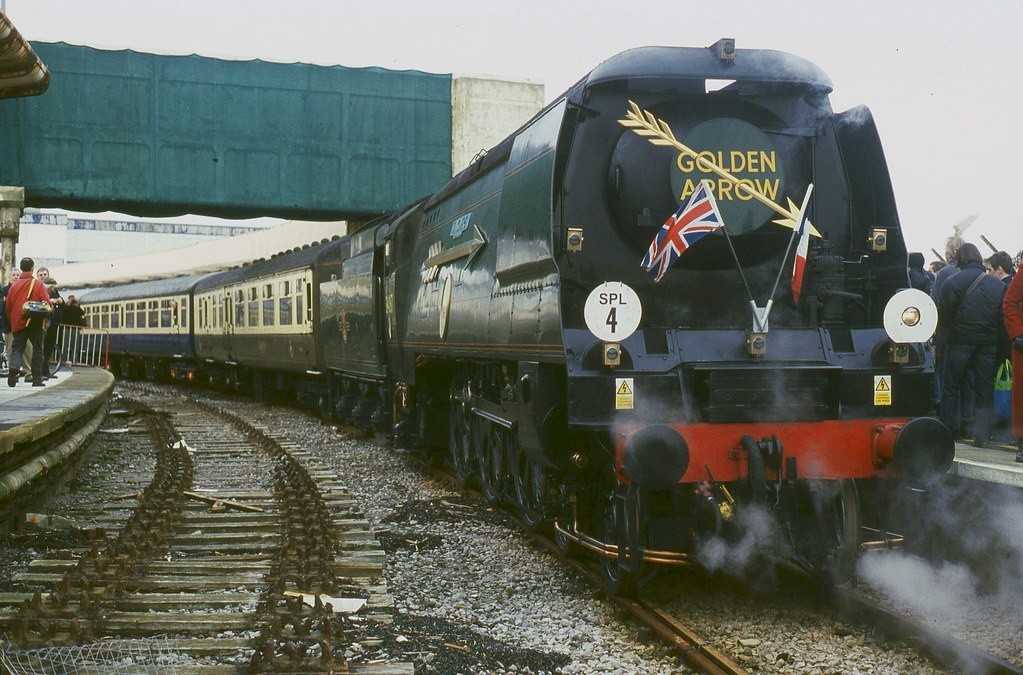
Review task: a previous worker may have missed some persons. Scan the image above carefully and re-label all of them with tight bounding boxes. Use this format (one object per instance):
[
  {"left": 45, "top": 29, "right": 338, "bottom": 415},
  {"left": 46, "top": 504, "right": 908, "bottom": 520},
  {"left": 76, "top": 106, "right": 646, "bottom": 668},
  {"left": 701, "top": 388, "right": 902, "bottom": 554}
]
[
  {"left": 0, "top": 257, "right": 85, "bottom": 387},
  {"left": 5, "top": 257, "right": 52, "bottom": 387},
  {"left": 910, "top": 243, "right": 1023, "bottom": 462}
]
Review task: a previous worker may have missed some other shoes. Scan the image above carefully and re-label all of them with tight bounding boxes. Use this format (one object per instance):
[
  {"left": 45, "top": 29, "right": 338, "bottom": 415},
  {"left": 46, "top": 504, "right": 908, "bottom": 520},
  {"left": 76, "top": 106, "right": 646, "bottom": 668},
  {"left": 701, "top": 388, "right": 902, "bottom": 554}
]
[
  {"left": 8, "top": 367, "right": 18, "bottom": 386},
  {"left": 41, "top": 375, "right": 49, "bottom": 380},
  {"left": 33, "top": 381, "right": 45, "bottom": 386},
  {"left": 45, "top": 373, "right": 58, "bottom": 378},
  {"left": 25, "top": 376, "right": 34, "bottom": 382},
  {"left": 1015, "top": 438, "right": 1023, "bottom": 462}
]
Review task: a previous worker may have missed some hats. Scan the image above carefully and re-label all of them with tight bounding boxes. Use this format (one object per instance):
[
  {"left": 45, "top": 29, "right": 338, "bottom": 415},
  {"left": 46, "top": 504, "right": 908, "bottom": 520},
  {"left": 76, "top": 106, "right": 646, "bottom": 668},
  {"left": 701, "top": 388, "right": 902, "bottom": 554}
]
[{"left": 44, "top": 277, "right": 57, "bottom": 284}]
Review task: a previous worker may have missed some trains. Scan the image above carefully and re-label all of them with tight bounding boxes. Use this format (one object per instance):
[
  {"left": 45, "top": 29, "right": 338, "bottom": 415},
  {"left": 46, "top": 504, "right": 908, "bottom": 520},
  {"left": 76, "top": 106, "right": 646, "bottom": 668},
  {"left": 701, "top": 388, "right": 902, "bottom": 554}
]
[{"left": 47, "top": 36, "right": 960, "bottom": 597}]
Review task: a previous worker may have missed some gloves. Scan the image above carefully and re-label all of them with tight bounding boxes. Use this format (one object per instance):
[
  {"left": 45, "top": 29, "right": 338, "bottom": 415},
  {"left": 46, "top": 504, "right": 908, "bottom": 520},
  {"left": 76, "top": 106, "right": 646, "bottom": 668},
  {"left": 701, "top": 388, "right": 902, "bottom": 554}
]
[{"left": 1014, "top": 336, "right": 1023, "bottom": 352}]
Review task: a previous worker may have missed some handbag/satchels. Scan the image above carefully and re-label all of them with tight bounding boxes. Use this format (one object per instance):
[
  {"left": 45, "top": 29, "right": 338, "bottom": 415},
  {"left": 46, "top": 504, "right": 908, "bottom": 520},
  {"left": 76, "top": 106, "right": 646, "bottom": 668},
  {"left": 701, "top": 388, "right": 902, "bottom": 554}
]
[
  {"left": 22, "top": 278, "right": 52, "bottom": 316},
  {"left": 992, "top": 359, "right": 1013, "bottom": 419}
]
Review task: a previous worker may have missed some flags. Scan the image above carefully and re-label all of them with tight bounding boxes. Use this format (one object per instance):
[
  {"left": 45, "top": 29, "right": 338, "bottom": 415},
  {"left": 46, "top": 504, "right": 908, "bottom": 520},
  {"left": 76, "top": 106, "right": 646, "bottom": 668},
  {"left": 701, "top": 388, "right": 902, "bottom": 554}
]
[
  {"left": 640, "top": 179, "right": 725, "bottom": 284},
  {"left": 791, "top": 183, "right": 813, "bottom": 305}
]
